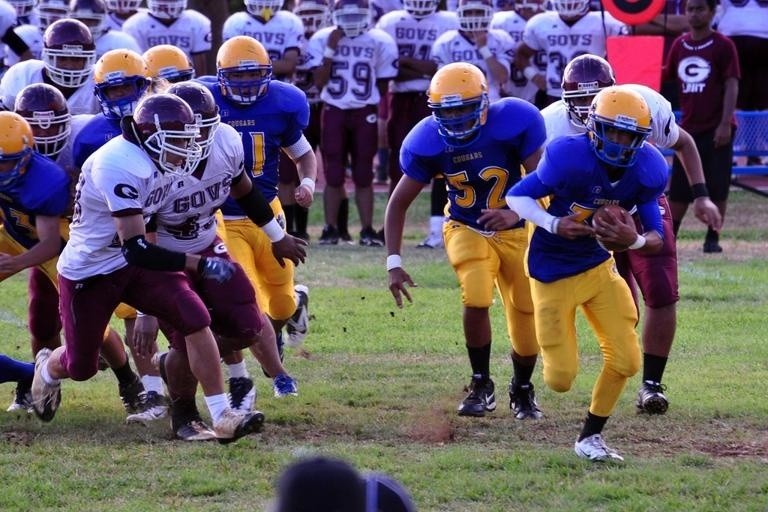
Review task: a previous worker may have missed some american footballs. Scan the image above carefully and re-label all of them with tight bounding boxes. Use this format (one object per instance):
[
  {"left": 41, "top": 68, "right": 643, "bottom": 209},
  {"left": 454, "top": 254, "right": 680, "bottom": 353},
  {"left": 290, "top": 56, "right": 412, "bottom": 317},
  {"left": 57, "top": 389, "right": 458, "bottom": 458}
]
[{"left": 592, "top": 205, "right": 637, "bottom": 252}]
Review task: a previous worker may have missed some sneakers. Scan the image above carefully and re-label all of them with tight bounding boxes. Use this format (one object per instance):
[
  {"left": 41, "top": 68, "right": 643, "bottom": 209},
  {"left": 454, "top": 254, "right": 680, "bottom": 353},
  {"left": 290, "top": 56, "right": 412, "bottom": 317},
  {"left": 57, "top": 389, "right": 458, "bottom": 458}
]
[
  {"left": 169, "top": 410, "right": 215, "bottom": 443},
  {"left": 635, "top": 380, "right": 671, "bottom": 413},
  {"left": 29, "top": 348, "right": 170, "bottom": 423},
  {"left": 224, "top": 374, "right": 258, "bottom": 416},
  {"left": 212, "top": 407, "right": 264, "bottom": 447},
  {"left": 296, "top": 225, "right": 445, "bottom": 248},
  {"left": 372, "top": 164, "right": 391, "bottom": 186},
  {"left": 572, "top": 433, "right": 626, "bottom": 463},
  {"left": 7, "top": 360, "right": 38, "bottom": 416},
  {"left": 455, "top": 373, "right": 496, "bottom": 417},
  {"left": 702, "top": 242, "right": 721, "bottom": 253},
  {"left": 262, "top": 285, "right": 309, "bottom": 402},
  {"left": 507, "top": 376, "right": 546, "bottom": 422}
]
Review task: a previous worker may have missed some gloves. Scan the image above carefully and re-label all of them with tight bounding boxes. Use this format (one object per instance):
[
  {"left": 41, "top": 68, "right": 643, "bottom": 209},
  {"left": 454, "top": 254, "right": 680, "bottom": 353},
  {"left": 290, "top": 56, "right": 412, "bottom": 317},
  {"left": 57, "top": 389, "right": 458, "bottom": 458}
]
[{"left": 196, "top": 255, "right": 235, "bottom": 284}]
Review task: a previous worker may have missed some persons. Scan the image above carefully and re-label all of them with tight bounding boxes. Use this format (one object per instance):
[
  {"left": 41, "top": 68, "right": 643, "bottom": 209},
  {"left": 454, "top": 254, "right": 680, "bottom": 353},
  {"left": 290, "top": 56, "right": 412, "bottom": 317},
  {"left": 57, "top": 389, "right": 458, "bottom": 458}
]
[{"left": 265, "top": 453, "right": 421, "bottom": 512}]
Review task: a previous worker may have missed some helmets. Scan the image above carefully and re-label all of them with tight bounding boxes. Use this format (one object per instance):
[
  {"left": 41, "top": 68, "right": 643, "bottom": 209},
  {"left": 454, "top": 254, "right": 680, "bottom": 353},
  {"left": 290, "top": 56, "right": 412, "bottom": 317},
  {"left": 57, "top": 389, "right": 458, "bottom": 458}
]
[
  {"left": 7, "top": 0, "right": 36, "bottom": 17},
  {"left": 550, "top": 0, "right": 589, "bottom": 18},
  {"left": 170, "top": 79, "right": 223, "bottom": 160},
  {"left": 103, "top": 0, "right": 143, "bottom": 15},
  {"left": 244, "top": 0, "right": 286, "bottom": 21},
  {"left": 513, "top": 0, "right": 548, "bottom": 13},
  {"left": 147, "top": 44, "right": 196, "bottom": 83},
  {"left": 148, "top": 0, "right": 187, "bottom": 19},
  {"left": 135, "top": 91, "right": 203, "bottom": 177},
  {"left": 15, "top": 83, "right": 72, "bottom": 157},
  {"left": 0, "top": 0, "right": 17, "bottom": 38},
  {"left": 403, "top": 0, "right": 440, "bottom": 19},
  {"left": 38, "top": 0, "right": 78, "bottom": 19},
  {"left": 331, "top": 0, "right": 373, "bottom": 37},
  {"left": 425, "top": 60, "right": 492, "bottom": 150},
  {"left": 455, "top": 0, "right": 493, "bottom": 31},
  {"left": 558, "top": 56, "right": 613, "bottom": 130},
  {"left": 216, "top": 34, "right": 275, "bottom": 107},
  {"left": 94, "top": 47, "right": 151, "bottom": 122},
  {"left": 292, "top": 0, "right": 330, "bottom": 35},
  {"left": 42, "top": 14, "right": 94, "bottom": 90},
  {"left": 0, "top": 111, "right": 34, "bottom": 191},
  {"left": 583, "top": 85, "right": 654, "bottom": 169}
]
